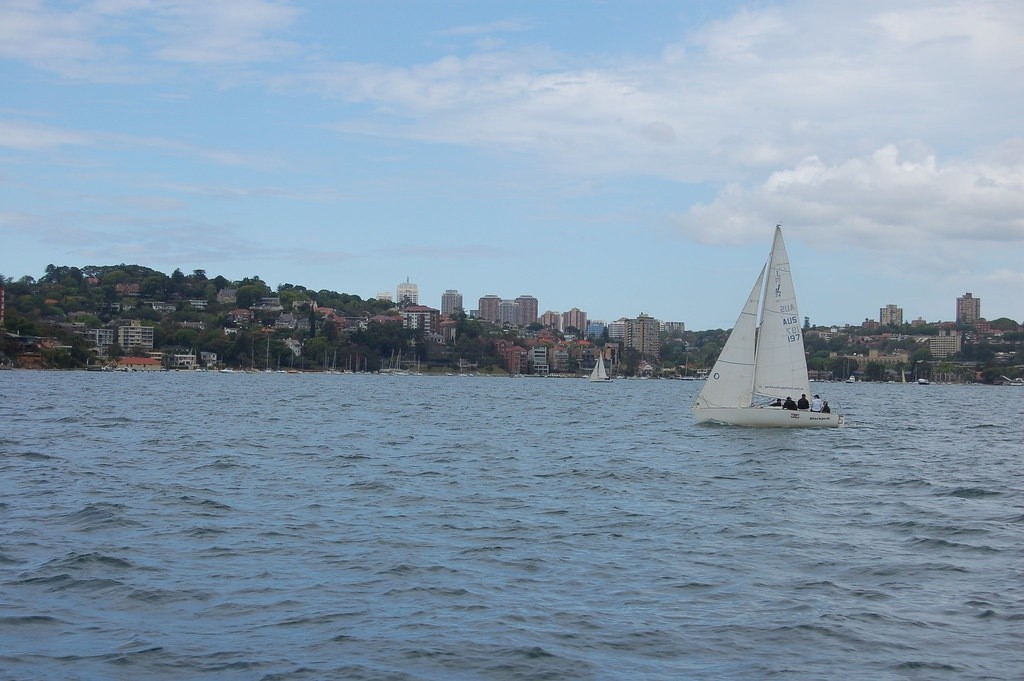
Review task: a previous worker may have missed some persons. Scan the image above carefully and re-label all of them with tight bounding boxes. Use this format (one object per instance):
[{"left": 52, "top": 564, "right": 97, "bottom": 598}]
[
  {"left": 782, "top": 397, "right": 797, "bottom": 410},
  {"left": 798, "top": 394, "right": 810, "bottom": 409},
  {"left": 810, "top": 394, "right": 823, "bottom": 413},
  {"left": 770, "top": 398, "right": 782, "bottom": 406},
  {"left": 821, "top": 401, "right": 830, "bottom": 413}
]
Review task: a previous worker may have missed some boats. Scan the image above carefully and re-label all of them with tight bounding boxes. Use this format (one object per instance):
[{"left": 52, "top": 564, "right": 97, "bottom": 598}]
[
  {"left": 617, "top": 374, "right": 708, "bottom": 381},
  {"left": 218, "top": 368, "right": 234, "bottom": 373},
  {"left": 917, "top": 378, "right": 930, "bottom": 385},
  {"left": 196, "top": 369, "right": 203, "bottom": 372},
  {"left": 845, "top": 376, "right": 856, "bottom": 383},
  {"left": 1011, "top": 377, "right": 1024, "bottom": 386}
]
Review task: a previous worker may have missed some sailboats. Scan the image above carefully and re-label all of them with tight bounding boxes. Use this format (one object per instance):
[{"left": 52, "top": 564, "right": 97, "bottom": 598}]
[
  {"left": 690, "top": 224, "right": 842, "bottom": 428},
  {"left": 238, "top": 336, "right": 308, "bottom": 374},
  {"left": 588, "top": 351, "right": 614, "bottom": 382},
  {"left": 323, "top": 347, "right": 423, "bottom": 376},
  {"left": 930, "top": 372, "right": 953, "bottom": 385}
]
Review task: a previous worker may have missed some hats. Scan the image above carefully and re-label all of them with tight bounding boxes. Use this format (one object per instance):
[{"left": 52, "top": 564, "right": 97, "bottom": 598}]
[
  {"left": 787, "top": 397, "right": 792, "bottom": 399},
  {"left": 813, "top": 395, "right": 820, "bottom": 398}
]
